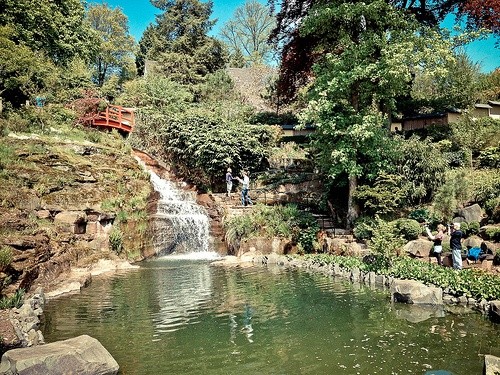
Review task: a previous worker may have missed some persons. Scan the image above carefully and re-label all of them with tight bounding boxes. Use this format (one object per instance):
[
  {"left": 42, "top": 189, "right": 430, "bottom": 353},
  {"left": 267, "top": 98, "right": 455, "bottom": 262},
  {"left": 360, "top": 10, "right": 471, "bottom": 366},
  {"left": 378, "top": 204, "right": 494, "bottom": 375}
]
[
  {"left": 425, "top": 224, "right": 446, "bottom": 267},
  {"left": 448, "top": 222, "right": 462, "bottom": 269},
  {"left": 34, "top": 88, "right": 46, "bottom": 109},
  {"left": 226, "top": 168, "right": 237, "bottom": 197},
  {"left": 236, "top": 171, "right": 255, "bottom": 206}
]
